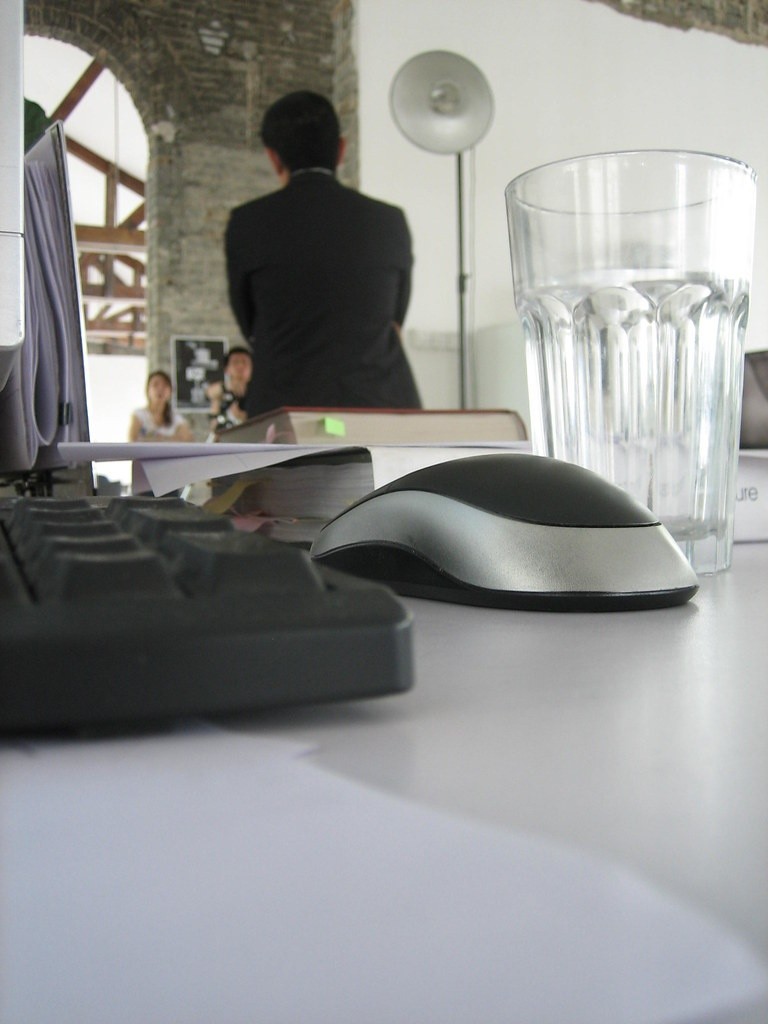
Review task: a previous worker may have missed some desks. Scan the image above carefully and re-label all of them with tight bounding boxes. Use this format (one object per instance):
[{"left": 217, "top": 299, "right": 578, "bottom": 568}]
[{"left": 0, "top": 453, "right": 768, "bottom": 1024}]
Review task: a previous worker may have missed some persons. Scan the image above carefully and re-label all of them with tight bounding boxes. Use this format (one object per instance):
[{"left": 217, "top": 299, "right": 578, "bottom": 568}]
[
  {"left": 223, "top": 89, "right": 424, "bottom": 418},
  {"left": 129, "top": 371, "right": 191, "bottom": 443},
  {"left": 206, "top": 344, "right": 254, "bottom": 443}
]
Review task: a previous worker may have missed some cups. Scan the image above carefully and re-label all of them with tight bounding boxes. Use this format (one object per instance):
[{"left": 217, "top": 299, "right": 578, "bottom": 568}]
[{"left": 504, "top": 148, "right": 759, "bottom": 571}]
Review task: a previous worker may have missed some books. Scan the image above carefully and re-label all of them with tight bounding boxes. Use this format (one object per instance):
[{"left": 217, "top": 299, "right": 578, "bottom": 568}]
[{"left": 210, "top": 405, "right": 531, "bottom": 543}]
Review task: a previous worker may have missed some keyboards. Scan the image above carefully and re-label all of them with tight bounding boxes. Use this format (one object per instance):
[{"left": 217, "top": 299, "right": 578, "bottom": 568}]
[{"left": 0, "top": 498, "right": 417, "bottom": 747}]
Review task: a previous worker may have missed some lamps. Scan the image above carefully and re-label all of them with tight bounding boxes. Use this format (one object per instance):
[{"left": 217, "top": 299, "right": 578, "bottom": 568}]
[{"left": 389, "top": 50, "right": 497, "bottom": 412}]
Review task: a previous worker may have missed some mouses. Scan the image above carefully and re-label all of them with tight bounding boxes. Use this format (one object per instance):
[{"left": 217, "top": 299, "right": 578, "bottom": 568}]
[{"left": 308, "top": 453, "right": 700, "bottom": 616}]
